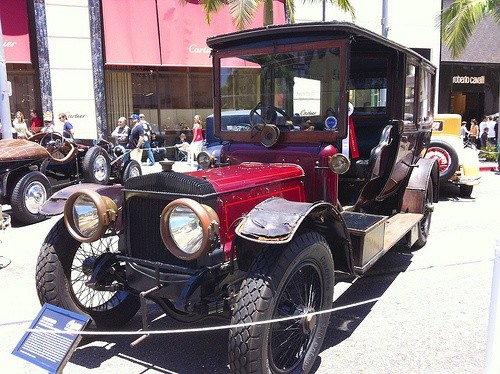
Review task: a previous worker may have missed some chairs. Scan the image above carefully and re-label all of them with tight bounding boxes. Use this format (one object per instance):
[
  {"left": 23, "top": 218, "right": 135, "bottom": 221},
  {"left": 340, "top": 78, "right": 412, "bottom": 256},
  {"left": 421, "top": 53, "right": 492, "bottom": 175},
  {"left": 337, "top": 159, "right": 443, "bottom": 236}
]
[{"left": 173, "top": 146, "right": 187, "bottom": 161}]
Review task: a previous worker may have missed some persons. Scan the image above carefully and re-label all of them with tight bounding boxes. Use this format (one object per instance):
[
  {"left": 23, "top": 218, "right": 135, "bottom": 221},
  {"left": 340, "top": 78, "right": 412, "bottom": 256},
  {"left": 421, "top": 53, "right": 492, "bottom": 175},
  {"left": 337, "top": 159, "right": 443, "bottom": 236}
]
[
  {"left": 175, "top": 132, "right": 190, "bottom": 160},
  {"left": 109, "top": 114, "right": 155, "bottom": 167},
  {"left": 13, "top": 108, "right": 76, "bottom": 144},
  {"left": 461, "top": 113, "right": 499, "bottom": 149},
  {"left": 187, "top": 114, "right": 204, "bottom": 165},
  {"left": 145, "top": 132, "right": 170, "bottom": 164}
]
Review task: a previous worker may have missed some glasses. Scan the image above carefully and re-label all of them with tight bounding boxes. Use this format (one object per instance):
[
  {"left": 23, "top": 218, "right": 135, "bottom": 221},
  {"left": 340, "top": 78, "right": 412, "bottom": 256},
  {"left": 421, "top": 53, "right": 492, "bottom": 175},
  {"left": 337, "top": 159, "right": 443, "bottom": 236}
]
[{"left": 59, "top": 117, "right": 63, "bottom": 119}]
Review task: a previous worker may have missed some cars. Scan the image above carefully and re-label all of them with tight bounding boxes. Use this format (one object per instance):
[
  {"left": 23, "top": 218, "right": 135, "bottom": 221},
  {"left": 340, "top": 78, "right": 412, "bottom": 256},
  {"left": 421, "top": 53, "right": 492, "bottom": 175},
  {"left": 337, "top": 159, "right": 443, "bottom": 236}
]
[
  {"left": 34, "top": 20, "right": 481, "bottom": 374},
  {"left": 0, "top": 125, "right": 142, "bottom": 225}
]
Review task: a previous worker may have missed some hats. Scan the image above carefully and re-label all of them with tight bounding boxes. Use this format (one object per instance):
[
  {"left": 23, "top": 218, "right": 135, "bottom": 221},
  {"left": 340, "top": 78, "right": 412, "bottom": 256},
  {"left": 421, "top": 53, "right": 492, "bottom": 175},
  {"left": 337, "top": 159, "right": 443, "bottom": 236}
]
[{"left": 128, "top": 114, "right": 139, "bottom": 119}]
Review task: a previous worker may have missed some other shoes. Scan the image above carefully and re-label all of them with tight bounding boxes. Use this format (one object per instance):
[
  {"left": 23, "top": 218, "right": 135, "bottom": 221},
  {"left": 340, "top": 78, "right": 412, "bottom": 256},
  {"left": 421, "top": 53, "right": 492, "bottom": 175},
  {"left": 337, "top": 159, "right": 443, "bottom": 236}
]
[{"left": 148, "top": 163, "right": 152, "bottom": 166}]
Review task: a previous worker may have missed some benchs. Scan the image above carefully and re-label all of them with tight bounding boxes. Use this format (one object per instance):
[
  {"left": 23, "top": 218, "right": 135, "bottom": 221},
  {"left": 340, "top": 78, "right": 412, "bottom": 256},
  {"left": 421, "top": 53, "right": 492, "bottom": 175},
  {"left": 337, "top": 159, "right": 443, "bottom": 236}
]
[{"left": 339, "top": 112, "right": 385, "bottom": 179}]
